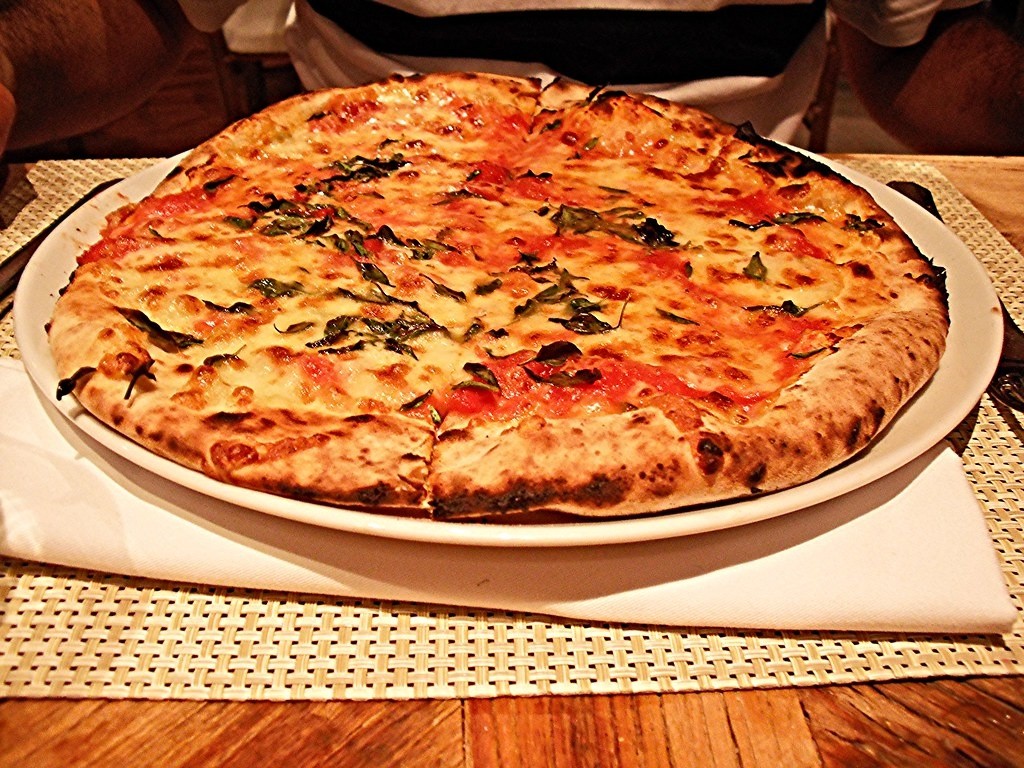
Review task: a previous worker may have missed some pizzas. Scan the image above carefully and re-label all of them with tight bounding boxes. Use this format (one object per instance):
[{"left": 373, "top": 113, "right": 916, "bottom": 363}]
[{"left": 48, "top": 71, "right": 952, "bottom": 517}]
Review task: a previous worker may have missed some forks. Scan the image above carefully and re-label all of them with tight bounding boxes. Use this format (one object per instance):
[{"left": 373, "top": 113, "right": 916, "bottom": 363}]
[{"left": 887, "top": 180, "right": 1024, "bottom": 414}]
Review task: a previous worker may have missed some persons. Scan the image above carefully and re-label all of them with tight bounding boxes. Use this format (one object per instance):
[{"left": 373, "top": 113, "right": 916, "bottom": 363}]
[{"left": 0, "top": 0, "right": 1024, "bottom": 164}]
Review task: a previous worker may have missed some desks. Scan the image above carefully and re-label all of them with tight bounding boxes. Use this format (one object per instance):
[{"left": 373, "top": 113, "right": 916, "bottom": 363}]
[{"left": 0, "top": 154, "right": 1024, "bottom": 768}]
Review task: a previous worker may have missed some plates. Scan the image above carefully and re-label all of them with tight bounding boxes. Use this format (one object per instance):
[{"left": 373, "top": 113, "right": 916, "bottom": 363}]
[{"left": 14, "top": 134, "right": 1005, "bottom": 547}]
